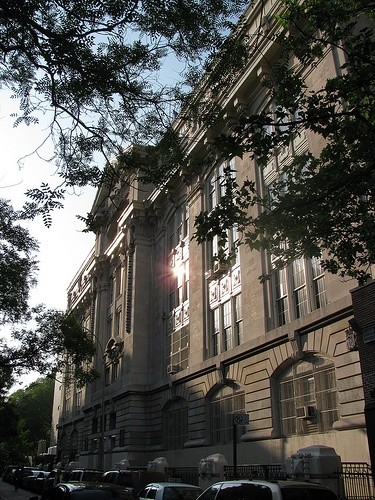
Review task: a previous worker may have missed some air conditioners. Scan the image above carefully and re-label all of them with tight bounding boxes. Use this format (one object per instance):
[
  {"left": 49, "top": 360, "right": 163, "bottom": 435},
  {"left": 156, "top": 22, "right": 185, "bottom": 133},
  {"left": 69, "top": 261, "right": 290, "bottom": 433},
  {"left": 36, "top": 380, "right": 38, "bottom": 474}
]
[
  {"left": 205, "top": 268, "right": 217, "bottom": 280},
  {"left": 213, "top": 262, "right": 224, "bottom": 274},
  {"left": 166, "top": 363, "right": 179, "bottom": 374},
  {"left": 296, "top": 406, "right": 317, "bottom": 421}
]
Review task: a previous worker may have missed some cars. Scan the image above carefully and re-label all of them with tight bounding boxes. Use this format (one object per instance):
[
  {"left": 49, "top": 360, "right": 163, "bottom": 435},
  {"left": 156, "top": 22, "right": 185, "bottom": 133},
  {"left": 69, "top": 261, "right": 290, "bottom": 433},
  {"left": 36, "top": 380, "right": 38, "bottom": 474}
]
[
  {"left": 2, "top": 465, "right": 165, "bottom": 500},
  {"left": 193, "top": 480, "right": 340, "bottom": 500},
  {"left": 136, "top": 482, "right": 201, "bottom": 500}
]
